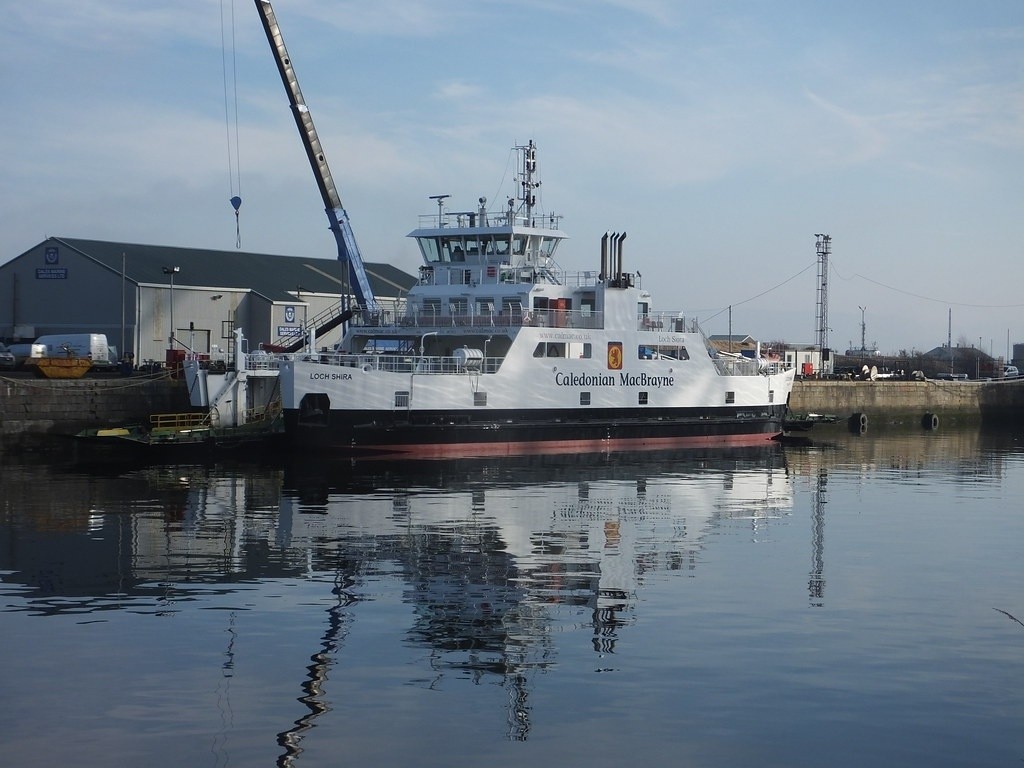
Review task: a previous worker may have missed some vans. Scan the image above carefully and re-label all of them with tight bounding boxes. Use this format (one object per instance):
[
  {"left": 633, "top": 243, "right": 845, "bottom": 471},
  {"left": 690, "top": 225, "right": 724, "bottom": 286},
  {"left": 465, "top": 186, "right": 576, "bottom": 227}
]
[
  {"left": 991, "top": 366, "right": 1019, "bottom": 380},
  {"left": 0, "top": 342, "right": 17, "bottom": 372},
  {"left": 6, "top": 344, "right": 47, "bottom": 368},
  {"left": 32, "top": 334, "right": 109, "bottom": 373}
]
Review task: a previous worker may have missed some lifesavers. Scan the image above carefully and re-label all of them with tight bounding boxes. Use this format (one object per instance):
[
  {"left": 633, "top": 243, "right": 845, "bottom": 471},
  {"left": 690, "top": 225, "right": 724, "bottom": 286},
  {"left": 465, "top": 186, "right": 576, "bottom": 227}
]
[
  {"left": 857, "top": 413, "right": 868, "bottom": 425},
  {"left": 928, "top": 414, "right": 939, "bottom": 429},
  {"left": 857, "top": 425, "right": 868, "bottom": 434}
]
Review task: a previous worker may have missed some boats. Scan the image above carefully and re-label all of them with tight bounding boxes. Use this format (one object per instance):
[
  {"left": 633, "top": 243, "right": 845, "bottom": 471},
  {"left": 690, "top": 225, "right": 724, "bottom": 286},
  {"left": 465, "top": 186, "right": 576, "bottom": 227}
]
[
  {"left": 279, "top": 139, "right": 798, "bottom": 465},
  {"left": 74, "top": 406, "right": 282, "bottom": 450}
]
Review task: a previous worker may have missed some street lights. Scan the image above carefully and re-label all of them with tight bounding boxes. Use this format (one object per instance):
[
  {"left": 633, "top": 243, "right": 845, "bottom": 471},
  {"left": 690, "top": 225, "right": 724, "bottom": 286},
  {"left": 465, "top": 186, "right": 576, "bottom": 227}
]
[
  {"left": 979, "top": 336, "right": 982, "bottom": 351},
  {"left": 162, "top": 264, "right": 180, "bottom": 349}
]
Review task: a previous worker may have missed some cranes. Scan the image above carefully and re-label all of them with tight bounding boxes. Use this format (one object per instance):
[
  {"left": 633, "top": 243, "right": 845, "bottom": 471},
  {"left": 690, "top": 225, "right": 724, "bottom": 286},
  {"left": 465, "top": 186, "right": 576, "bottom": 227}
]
[{"left": 222, "top": 1, "right": 380, "bottom": 321}]
